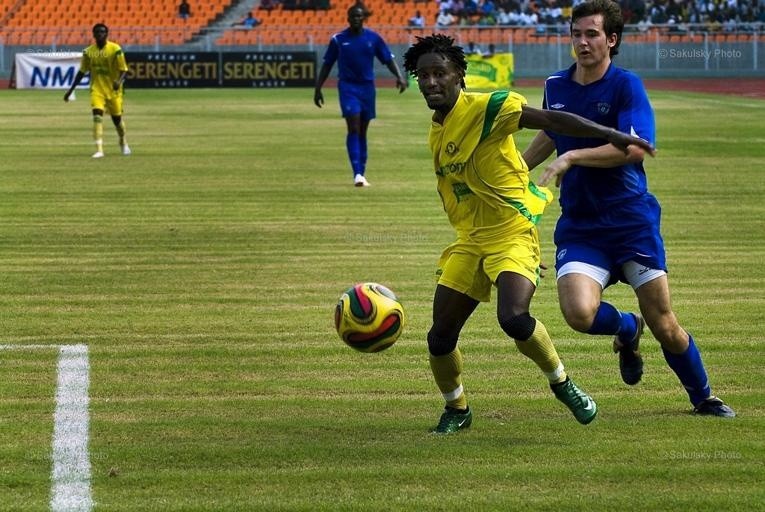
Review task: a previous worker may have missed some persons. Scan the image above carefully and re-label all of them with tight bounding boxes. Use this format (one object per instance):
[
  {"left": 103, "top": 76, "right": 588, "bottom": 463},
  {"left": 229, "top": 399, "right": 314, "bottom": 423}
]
[
  {"left": 522, "top": 2, "right": 735, "bottom": 418},
  {"left": 231, "top": 12, "right": 259, "bottom": 28},
  {"left": 64, "top": 24, "right": 131, "bottom": 158},
  {"left": 408, "top": 10, "right": 425, "bottom": 26},
  {"left": 179, "top": 0, "right": 190, "bottom": 18},
  {"left": 313, "top": 5, "right": 410, "bottom": 186},
  {"left": 404, "top": 34, "right": 654, "bottom": 432}
]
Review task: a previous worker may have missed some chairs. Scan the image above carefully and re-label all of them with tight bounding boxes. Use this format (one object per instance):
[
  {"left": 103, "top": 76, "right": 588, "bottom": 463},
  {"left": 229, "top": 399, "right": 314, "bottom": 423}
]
[{"left": 1, "top": 0, "right": 765, "bottom": 49}]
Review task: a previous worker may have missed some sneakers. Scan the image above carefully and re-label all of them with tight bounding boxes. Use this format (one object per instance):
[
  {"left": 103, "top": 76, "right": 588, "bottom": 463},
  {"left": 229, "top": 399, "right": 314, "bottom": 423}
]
[
  {"left": 693, "top": 395, "right": 737, "bottom": 417},
  {"left": 613, "top": 312, "right": 645, "bottom": 385},
  {"left": 92, "top": 151, "right": 103, "bottom": 158},
  {"left": 550, "top": 374, "right": 598, "bottom": 425},
  {"left": 118, "top": 140, "right": 130, "bottom": 155},
  {"left": 362, "top": 176, "right": 370, "bottom": 187},
  {"left": 354, "top": 173, "right": 363, "bottom": 186},
  {"left": 434, "top": 405, "right": 472, "bottom": 434}
]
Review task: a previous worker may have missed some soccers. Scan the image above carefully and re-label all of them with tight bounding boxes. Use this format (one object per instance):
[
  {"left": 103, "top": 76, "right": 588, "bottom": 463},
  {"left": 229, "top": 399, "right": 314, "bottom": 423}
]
[{"left": 335, "top": 283, "right": 405, "bottom": 353}]
